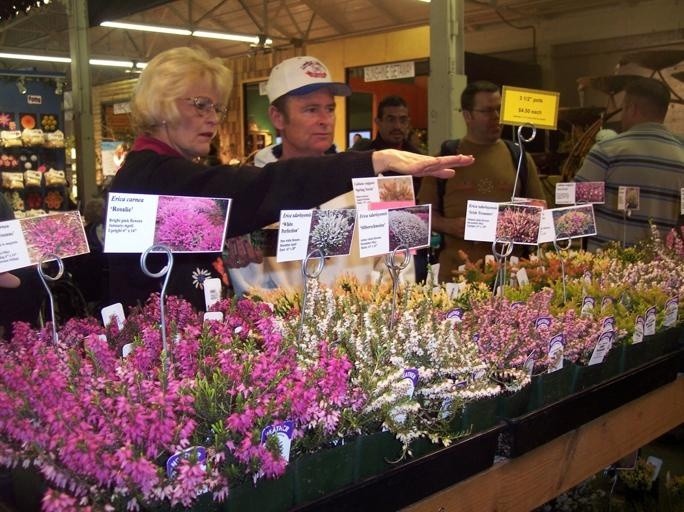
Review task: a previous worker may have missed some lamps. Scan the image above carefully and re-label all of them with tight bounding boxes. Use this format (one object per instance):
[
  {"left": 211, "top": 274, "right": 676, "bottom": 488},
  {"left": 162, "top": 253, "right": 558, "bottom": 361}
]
[
  {"left": 1, "top": 73, "right": 66, "bottom": 96},
  {"left": 89, "top": 59, "right": 147, "bottom": 70},
  {"left": 0, "top": 53, "right": 72, "bottom": 64},
  {"left": 99, "top": 20, "right": 273, "bottom": 50},
  {"left": 0, "top": 0, "right": 51, "bottom": 22}
]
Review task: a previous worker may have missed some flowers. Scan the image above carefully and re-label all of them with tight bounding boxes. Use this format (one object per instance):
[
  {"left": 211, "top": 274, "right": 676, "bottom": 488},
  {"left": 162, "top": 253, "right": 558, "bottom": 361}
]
[{"left": 1, "top": 217, "right": 684, "bottom": 511}]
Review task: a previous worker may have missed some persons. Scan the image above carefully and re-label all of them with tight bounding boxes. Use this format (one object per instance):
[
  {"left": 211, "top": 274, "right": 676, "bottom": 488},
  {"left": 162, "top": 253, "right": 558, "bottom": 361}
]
[{"left": 1, "top": 48, "right": 684, "bottom": 336}]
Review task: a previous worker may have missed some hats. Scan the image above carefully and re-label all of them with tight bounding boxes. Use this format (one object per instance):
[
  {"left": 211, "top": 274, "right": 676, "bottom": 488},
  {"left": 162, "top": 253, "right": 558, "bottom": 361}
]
[{"left": 266, "top": 55, "right": 352, "bottom": 104}]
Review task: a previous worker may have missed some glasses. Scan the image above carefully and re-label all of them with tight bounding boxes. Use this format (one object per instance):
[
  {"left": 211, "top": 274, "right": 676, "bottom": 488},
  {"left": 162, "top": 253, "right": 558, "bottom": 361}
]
[
  {"left": 177, "top": 96, "right": 228, "bottom": 124},
  {"left": 470, "top": 108, "right": 502, "bottom": 115},
  {"left": 382, "top": 117, "right": 414, "bottom": 128}
]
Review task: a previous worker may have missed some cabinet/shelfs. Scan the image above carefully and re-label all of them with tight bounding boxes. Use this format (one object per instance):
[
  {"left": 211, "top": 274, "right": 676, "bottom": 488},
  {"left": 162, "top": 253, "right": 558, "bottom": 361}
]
[{"left": 247, "top": 131, "right": 267, "bottom": 164}]
